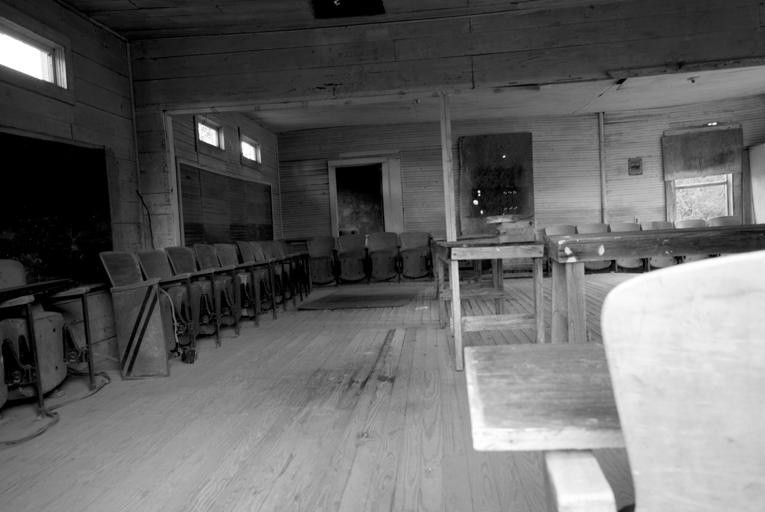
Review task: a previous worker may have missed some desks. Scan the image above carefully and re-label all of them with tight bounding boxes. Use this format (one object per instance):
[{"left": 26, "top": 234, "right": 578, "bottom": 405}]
[
  {"left": 459, "top": 338, "right": 663, "bottom": 456},
  {"left": 425, "top": 231, "right": 507, "bottom": 329},
  {"left": 441, "top": 240, "right": 549, "bottom": 372},
  {"left": 540, "top": 221, "right": 762, "bottom": 344}
]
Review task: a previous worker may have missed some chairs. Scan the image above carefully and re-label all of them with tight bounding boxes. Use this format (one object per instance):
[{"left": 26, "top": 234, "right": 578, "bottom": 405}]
[
  {"left": 0, "top": 216, "right": 318, "bottom": 430},
  {"left": 306, "top": 216, "right": 762, "bottom": 281}
]
